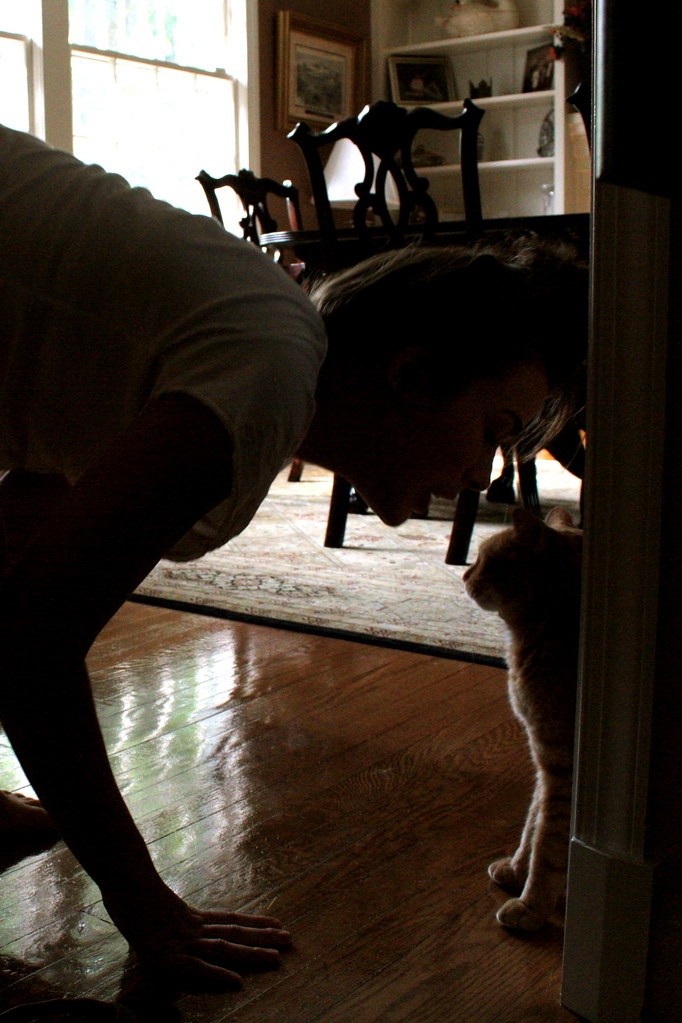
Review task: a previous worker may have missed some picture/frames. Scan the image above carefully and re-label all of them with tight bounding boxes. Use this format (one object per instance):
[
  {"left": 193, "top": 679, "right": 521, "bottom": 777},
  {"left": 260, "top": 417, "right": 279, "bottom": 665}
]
[
  {"left": 276, "top": 10, "right": 368, "bottom": 134},
  {"left": 387, "top": 57, "right": 456, "bottom": 105}
]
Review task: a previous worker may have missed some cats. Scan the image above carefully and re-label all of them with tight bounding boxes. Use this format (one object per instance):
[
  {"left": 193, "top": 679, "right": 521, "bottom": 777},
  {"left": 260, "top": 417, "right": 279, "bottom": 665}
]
[{"left": 460, "top": 483, "right": 592, "bottom": 928}]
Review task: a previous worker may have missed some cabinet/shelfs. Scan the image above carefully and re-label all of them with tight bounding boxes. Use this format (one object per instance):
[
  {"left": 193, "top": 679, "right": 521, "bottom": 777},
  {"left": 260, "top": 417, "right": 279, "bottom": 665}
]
[{"left": 370, "top": 0, "right": 568, "bottom": 220}]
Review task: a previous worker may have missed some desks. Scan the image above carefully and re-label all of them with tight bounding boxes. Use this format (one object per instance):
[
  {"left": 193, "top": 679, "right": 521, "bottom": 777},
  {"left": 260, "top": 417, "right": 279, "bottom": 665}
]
[{"left": 259, "top": 212, "right": 592, "bottom": 512}]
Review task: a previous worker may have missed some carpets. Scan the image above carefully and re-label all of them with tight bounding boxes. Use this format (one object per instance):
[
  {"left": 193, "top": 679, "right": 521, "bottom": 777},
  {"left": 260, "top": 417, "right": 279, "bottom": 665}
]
[{"left": 132, "top": 457, "right": 582, "bottom": 665}]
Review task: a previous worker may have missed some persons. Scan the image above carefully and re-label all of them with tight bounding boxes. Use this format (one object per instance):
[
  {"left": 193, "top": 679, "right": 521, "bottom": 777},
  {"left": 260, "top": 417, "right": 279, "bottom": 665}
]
[
  {"left": 410, "top": 71, "right": 426, "bottom": 98},
  {"left": 0, "top": 127, "right": 582, "bottom": 992},
  {"left": 531, "top": 55, "right": 553, "bottom": 90}
]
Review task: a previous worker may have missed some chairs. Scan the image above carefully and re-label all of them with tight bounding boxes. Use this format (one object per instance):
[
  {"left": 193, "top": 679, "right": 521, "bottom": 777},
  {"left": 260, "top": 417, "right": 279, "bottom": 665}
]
[{"left": 196, "top": 98, "right": 484, "bottom": 565}]
